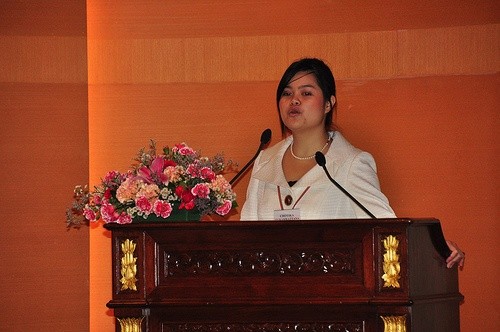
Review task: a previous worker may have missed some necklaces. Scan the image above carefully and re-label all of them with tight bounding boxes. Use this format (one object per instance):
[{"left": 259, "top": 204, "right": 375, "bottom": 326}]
[{"left": 290, "top": 134, "right": 331, "bottom": 160}]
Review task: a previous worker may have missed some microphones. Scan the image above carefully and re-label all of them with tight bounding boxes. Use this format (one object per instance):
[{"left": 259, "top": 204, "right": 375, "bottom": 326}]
[
  {"left": 315, "top": 151, "right": 377, "bottom": 219},
  {"left": 228, "top": 127, "right": 272, "bottom": 187}
]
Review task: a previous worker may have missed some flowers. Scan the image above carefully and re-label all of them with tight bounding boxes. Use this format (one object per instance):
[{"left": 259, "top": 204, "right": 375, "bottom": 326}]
[{"left": 62, "top": 138, "right": 240, "bottom": 230}]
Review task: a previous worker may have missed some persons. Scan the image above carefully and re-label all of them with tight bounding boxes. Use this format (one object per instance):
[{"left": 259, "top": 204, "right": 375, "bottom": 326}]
[{"left": 240, "top": 58, "right": 465, "bottom": 271}]
